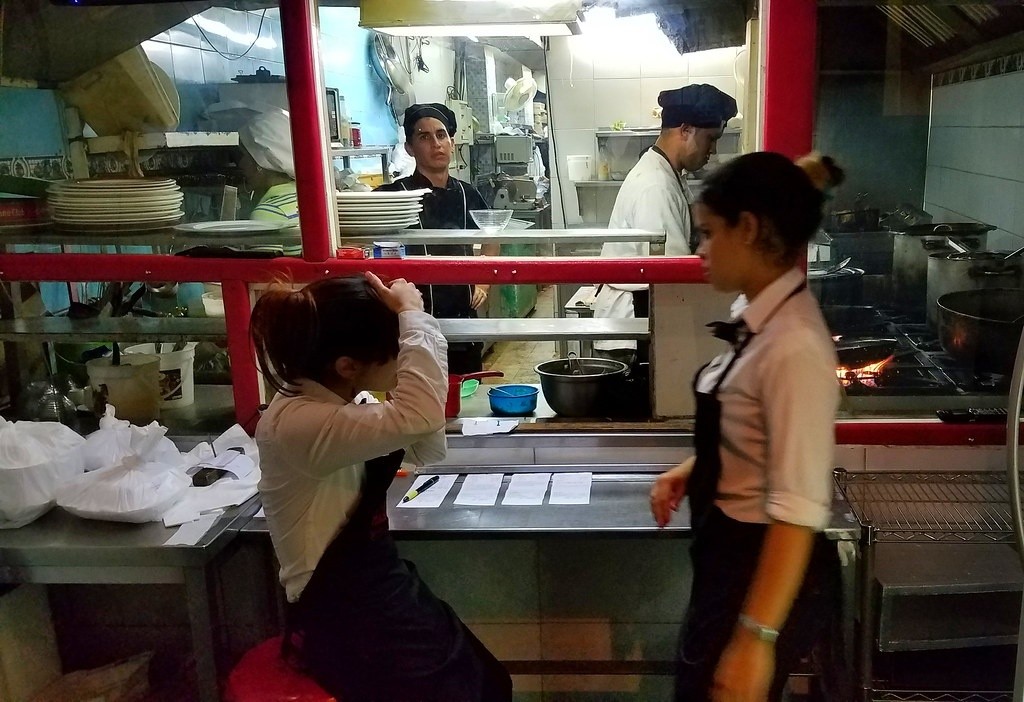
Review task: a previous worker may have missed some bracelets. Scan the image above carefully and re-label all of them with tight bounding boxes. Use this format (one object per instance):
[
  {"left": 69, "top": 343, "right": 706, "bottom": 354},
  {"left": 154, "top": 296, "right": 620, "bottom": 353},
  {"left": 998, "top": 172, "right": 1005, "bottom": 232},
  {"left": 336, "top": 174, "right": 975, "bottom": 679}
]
[{"left": 736, "top": 614, "right": 778, "bottom": 644}]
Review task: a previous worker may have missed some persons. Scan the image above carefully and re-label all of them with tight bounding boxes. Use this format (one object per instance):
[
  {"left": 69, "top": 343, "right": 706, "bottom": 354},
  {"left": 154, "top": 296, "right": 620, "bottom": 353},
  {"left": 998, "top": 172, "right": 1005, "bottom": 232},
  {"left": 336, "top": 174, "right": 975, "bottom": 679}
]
[
  {"left": 236, "top": 107, "right": 302, "bottom": 257},
  {"left": 247, "top": 271, "right": 513, "bottom": 702},
  {"left": 594, "top": 84, "right": 737, "bottom": 363},
  {"left": 371, "top": 104, "right": 500, "bottom": 385},
  {"left": 648, "top": 152, "right": 845, "bottom": 702}
]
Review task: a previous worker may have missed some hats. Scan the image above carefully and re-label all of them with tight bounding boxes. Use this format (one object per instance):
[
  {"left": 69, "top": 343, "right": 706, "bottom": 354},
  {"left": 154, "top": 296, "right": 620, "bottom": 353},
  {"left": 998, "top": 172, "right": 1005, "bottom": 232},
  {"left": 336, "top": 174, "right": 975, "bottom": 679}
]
[
  {"left": 658, "top": 83, "right": 738, "bottom": 128},
  {"left": 404, "top": 103, "right": 458, "bottom": 142},
  {"left": 239, "top": 107, "right": 296, "bottom": 180}
]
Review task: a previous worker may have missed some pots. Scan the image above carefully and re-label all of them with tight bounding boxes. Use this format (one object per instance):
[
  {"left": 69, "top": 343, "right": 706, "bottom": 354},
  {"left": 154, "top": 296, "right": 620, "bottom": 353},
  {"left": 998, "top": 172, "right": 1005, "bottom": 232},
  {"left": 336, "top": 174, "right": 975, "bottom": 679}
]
[
  {"left": 891, "top": 222, "right": 997, "bottom": 300},
  {"left": 927, "top": 251, "right": 1024, "bottom": 336},
  {"left": 936, "top": 289, "right": 1024, "bottom": 361},
  {"left": 385, "top": 368, "right": 504, "bottom": 416}
]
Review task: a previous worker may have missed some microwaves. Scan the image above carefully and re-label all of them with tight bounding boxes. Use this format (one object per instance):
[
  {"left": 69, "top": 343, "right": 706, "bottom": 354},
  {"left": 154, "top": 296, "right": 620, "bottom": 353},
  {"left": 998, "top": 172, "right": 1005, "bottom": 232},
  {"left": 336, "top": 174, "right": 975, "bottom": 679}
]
[{"left": 218, "top": 84, "right": 345, "bottom": 147}]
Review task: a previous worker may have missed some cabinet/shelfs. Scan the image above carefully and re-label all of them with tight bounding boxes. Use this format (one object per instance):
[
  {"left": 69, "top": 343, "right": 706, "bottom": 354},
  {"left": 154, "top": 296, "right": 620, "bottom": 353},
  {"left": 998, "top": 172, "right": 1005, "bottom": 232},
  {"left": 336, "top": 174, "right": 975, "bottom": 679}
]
[
  {"left": 0, "top": 491, "right": 261, "bottom": 702},
  {"left": 0, "top": 232, "right": 666, "bottom": 436},
  {"left": 573, "top": 128, "right": 742, "bottom": 216}
]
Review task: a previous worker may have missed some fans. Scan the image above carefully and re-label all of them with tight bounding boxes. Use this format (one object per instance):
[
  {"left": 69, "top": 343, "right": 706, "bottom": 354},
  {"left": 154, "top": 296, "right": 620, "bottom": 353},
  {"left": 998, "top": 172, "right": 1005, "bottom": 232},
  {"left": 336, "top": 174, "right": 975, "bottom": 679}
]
[{"left": 492, "top": 77, "right": 537, "bottom": 121}]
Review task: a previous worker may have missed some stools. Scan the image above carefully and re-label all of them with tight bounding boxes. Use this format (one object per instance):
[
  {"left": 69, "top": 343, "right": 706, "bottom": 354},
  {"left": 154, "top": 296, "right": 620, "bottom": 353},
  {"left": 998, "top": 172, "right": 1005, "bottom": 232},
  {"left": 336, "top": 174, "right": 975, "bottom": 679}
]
[{"left": 226, "top": 630, "right": 337, "bottom": 702}]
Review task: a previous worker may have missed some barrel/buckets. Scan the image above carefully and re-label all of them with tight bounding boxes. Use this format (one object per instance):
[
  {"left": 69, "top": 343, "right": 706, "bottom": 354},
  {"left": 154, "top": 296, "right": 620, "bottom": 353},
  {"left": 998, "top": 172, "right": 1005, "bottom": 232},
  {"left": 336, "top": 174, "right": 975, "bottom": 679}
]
[
  {"left": 52, "top": 341, "right": 118, "bottom": 376},
  {"left": 124, "top": 344, "right": 196, "bottom": 408},
  {"left": 567, "top": 155, "right": 592, "bottom": 181},
  {"left": 87, "top": 357, "right": 161, "bottom": 422}
]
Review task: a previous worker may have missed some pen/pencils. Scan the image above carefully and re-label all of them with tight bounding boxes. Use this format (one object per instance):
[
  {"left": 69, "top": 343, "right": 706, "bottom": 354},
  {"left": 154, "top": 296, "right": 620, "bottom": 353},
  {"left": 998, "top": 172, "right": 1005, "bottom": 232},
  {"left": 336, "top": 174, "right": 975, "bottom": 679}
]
[{"left": 403, "top": 475, "right": 440, "bottom": 503}]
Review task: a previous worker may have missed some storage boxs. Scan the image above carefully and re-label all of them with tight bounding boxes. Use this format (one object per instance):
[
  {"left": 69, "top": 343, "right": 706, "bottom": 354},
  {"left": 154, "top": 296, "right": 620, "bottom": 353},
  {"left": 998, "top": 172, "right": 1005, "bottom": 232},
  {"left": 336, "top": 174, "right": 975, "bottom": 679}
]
[{"left": 0, "top": 583, "right": 153, "bottom": 701}]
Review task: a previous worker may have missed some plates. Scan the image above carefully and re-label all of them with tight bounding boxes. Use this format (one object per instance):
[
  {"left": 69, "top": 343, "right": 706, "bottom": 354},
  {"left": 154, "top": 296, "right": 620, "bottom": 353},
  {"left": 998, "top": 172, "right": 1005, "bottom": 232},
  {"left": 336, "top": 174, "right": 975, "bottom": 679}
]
[
  {"left": 88, "top": 59, "right": 180, "bottom": 161},
  {"left": 336, "top": 189, "right": 424, "bottom": 233},
  {"left": 48, "top": 178, "right": 183, "bottom": 225},
  {"left": 175, "top": 220, "right": 293, "bottom": 232}
]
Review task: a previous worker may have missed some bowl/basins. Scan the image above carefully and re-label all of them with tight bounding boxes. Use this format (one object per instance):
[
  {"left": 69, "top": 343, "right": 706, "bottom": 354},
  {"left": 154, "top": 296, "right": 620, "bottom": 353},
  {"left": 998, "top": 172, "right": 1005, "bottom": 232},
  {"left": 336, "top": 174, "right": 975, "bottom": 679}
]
[
  {"left": 488, "top": 385, "right": 539, "bottom": 413},
  {"left": 461, "top": 380, "right": 479, "bottom": 398},
  {"left": 534, "top": 358, "right": 628, "bottom": 416},
  {"left": 611, "top": 171, "right": 628, "bottom": 180},
  {"left": 469, "top": 210, "right": 514, "bottom": 233}
]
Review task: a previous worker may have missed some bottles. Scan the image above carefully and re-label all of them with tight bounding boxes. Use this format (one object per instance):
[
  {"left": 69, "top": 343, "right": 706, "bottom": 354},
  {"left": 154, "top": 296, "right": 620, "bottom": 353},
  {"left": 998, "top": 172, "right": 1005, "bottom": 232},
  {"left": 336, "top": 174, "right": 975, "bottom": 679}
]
[
  {"left": 350, "top": 121, "right": 361, "bottom": 148},
  {"left": 339, "top": 97, "right": 351, "bottom": 147}
]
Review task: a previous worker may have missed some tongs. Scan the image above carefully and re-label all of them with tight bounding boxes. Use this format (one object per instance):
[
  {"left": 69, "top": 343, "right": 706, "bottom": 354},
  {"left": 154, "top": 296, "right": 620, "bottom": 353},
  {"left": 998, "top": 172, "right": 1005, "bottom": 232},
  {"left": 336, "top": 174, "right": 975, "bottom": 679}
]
[{"left": 945, "top": 235, "right": 972, "bottom": 254}]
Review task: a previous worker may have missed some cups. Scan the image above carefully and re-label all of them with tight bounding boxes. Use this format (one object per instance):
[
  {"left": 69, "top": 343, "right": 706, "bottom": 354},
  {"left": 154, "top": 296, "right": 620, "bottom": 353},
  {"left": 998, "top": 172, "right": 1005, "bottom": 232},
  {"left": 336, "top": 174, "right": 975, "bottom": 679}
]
[{"left": 202, "top": 292, "right": 223, "bottom": 318}]
